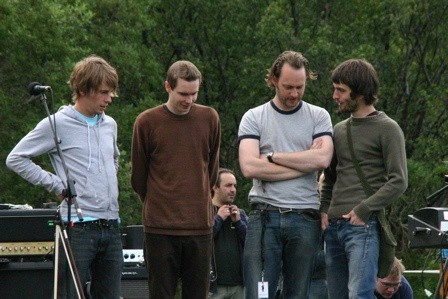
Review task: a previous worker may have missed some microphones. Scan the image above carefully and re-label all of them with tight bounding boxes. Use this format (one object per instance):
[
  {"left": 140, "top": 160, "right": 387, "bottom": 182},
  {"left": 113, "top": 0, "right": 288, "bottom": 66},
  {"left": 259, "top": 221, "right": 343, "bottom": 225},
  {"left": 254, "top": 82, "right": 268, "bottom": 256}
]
[{"left": 28, "top": 81, "right": 50, "bottom": 94}]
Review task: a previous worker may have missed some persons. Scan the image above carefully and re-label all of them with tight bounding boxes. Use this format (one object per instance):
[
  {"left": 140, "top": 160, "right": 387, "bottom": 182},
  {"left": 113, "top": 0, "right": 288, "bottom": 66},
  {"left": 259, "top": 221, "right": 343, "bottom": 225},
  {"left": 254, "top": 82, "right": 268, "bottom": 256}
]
[
  {"left": 207, "top": 168, "right": 248, "bottom": 299},
  {"left": 131, "top": 61, "right": 221, "bottom": 299},
  {"left": 237, "top": 51, "right": 334, "bottom": 299},
  {"left": 376, "top": 256, "right": 414, "bottom": 299},
  {"left": 318, "top": 59, "right": 407, "bottom": 299},
  {"left": 6, "top": 55, "right": 123, "bottom": 299}
]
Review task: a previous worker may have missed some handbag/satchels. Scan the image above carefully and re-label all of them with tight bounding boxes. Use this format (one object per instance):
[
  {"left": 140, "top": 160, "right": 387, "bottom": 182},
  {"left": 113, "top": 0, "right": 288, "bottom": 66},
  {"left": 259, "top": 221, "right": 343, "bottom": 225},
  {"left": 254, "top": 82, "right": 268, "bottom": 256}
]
[{"left": 377, "top": 221, "right": 407, "bottom": 279}]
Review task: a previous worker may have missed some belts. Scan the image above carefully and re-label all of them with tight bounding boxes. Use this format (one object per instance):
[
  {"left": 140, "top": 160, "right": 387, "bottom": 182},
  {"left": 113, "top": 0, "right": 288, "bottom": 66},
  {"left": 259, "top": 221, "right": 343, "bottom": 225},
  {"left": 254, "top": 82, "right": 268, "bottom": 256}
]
[{"left": 253, "top": 204, "right": 316, "bottom": 214}]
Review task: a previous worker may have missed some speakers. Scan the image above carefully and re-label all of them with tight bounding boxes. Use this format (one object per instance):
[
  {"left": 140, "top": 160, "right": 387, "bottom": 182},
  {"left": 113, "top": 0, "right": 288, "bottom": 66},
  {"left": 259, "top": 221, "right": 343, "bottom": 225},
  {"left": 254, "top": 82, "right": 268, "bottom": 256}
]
[
  {"left": 408, "top": 207, "right": 448, "bottom": 248},
  {"left": 121, "top": 268, "right": 150, "bottom": 299},
  {"left": 0, "top": 259, "right": 55, "bottom": 299}
]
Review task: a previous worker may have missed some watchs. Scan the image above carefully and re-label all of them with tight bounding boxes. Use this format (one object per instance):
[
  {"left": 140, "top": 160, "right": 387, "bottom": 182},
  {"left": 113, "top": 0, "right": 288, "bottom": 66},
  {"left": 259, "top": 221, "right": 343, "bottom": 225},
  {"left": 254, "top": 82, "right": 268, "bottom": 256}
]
[{"left": 267, "top": 152, "right": 274, "bottom": 162}]
